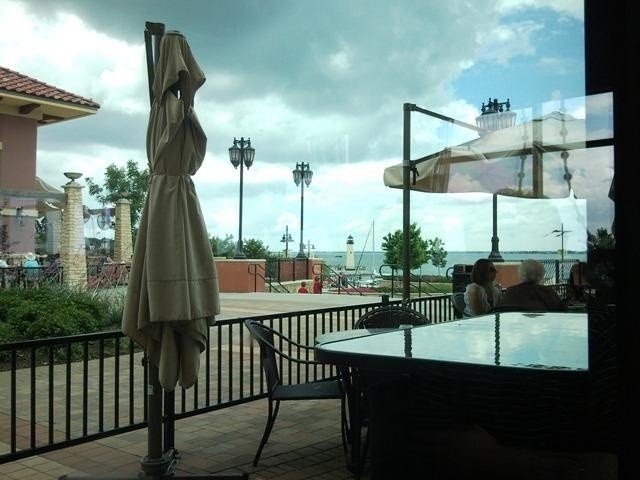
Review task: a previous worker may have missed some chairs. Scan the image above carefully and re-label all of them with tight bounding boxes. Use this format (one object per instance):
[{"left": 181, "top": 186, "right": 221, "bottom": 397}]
[
  {"left": 349, "top": 306, "right": 434, "bottom": 473},
  {"left": 0, "top": 258, "right": 130, "bottom": 293},
  {"left": 450, "top": 291, "right": 466, "bottom": 320},
  {"left": 242, "top": 318, "right": 364, "bottom": 469}
]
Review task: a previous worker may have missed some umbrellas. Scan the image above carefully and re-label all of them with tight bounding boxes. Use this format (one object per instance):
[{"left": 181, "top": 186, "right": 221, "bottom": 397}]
[
  {"left": 120, "top": 19, "right": 224, "bottom": 480},
  {"left": 385, "top": 108, "right": 619, "bottom": 200}
]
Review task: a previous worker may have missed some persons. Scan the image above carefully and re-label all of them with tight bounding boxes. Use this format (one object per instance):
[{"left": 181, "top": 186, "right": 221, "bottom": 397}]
[
  {"left": 296, "top": 282, "right": 309, "bottom": 293},
  {"left": 0, "top": 250, "right": 63, "bottom": 288},
  {"left": 312, "top": 276, "right": 323, "bottom": 295},
  {"left": 463, "top": 257, "right": 503, "bottom": 318},
  {"left": 502, "top": 258, "right": 568, "bottom": 313}
]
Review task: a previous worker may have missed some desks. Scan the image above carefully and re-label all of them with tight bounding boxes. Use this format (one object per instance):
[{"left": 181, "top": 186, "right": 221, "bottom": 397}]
[
  {"left": 314, "top": 325, "right": 406, "bottom": 465},
  {"left": 316, "top": 306, "right": 592, "bottom": 480}
]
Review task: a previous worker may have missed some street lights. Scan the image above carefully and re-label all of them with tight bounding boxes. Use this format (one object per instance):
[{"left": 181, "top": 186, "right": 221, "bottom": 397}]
[
  {"left": 279, "top": 225, "right": 294, "bottom": 258},
  {"left": 227, "top": 136, "right": 255, "bottom": 259},
  {"left": 552, "top": 223, "right": 574, "bottom": 281},
  {"left": 292, "top": 161, "right": 313, "bottom": 259},
  {"left": 303, "top": 240, "right": 317, "bottom": 257},
  {"left": 476, "top": 97, "right": 517, "bottom": 263}
]
